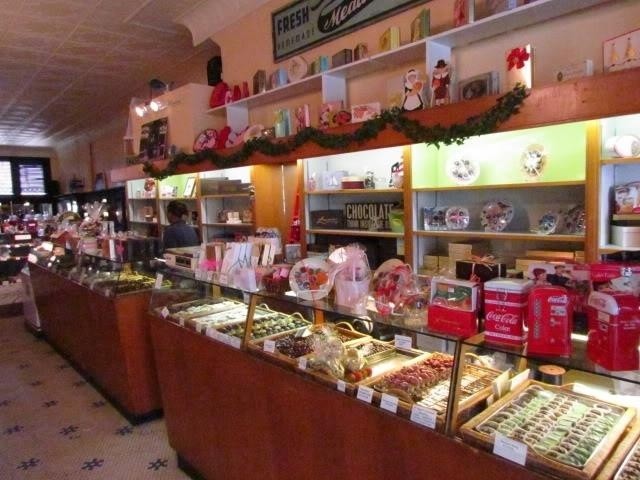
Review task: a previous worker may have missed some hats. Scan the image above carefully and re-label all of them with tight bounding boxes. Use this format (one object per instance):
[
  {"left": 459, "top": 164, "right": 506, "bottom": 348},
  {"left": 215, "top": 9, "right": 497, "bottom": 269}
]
[{"left": 167, "top": 201, "right": 189, "bottom": 218}]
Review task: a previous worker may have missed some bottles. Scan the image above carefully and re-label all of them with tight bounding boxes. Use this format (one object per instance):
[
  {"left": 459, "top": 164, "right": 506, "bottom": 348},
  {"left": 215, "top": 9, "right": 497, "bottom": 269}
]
[
  {"left": 613, "top": 180, "right": 640, "bottom": 214},
  {"left": 224, "top": 80, "right": 250, "bottom": 107}
]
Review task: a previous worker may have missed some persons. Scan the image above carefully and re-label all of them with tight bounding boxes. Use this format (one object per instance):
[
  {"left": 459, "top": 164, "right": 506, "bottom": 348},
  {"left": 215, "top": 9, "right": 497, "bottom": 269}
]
[
  {"left": 401, "top": 69, "right": 424, "bottom": 111},
  {"left": 547, "top": 265, "right": 572, "bottom": 287},
  {"left": 160, "top": 200, "right": 199, "bottom": 252},
  {"left": 429, "top": 58, "right": 452, "bottom": 108},
  {"left": 532, "top": 268, "right": 547, "bottom": 284}
]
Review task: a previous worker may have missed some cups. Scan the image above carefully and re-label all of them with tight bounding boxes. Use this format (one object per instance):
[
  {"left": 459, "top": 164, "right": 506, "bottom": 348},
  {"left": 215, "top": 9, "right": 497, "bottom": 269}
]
[
  {"left": 215, "top": 207, "right": 253, "bottom": 224},
  {"left": 96, "top": 231, "right": 160, "bottom": 261},
  {"left": 391, "top": 218, "right": 403, "bottom": 233},
  {"left": 286, "top": 245, "right": 302, "bottom": 264},
  {"left": 144, "top": 206, "right": 154, "bottom": 222}
]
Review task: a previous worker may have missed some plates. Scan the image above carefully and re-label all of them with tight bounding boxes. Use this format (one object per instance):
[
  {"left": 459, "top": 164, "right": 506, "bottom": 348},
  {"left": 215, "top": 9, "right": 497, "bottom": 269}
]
[
  {"left": 193, "top": 124, "right": 264, "bottom": 153},
  {"left": 209, "top": 79, "right": 228, "bottom": 109},
  {"left": 288, "top": 258, "right": 335, "bottom": 301},
  {"left": 446, "top": 207, "right": 471, "bottom": 230},
  {"left": 446, "top": 155, "right": 480, "bottom": 185},
  {"left": 519, "top": 143, "right": 547, "bottom": 182},
  {"left": 537, "top": 212, "right": 559, "bottom": 236},
  {"left": 342, "top": 182, "right": 365, "bottom": 189},
  {"left": 565, "top": 206, "right": 587, "bottom": 236},
  {"left": 479, "top": 200, "right": 514, "bottom": 232},
  {"left": 310, "top": 170, "right": 349, "bottom": 188}
]
